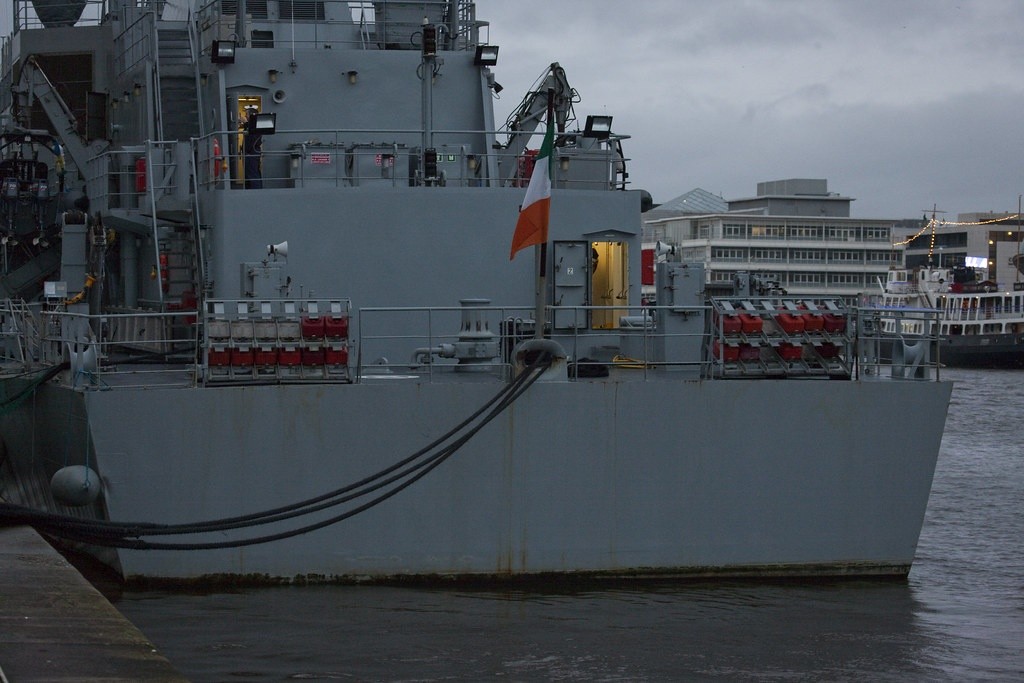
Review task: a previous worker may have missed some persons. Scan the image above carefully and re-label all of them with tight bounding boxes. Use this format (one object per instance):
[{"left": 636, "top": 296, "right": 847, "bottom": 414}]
[{"left": 238, "top": 109, "right": 263, "bottom": 189}]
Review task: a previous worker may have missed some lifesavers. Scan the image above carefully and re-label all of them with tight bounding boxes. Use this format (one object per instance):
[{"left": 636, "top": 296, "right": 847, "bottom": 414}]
[
  {"left": 159, "top": 254, "right": 168, "bottom": 292},
  {"left": 208, "top": 138, "right": 220, "bottom": 177}
]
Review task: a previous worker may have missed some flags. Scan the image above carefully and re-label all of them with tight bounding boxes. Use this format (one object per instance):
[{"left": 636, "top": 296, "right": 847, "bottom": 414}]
[{"left": 509, "top": 111, "right": 555, "bottom": 262}]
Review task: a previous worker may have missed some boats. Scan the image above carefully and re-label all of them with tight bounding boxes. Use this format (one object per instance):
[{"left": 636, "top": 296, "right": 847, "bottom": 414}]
[
  {"left": 854, "top": 245, "right": 1024, "bottom": 369},
  {"left": 1, "top": 1, "right": 958, "bottom": 596}
]
